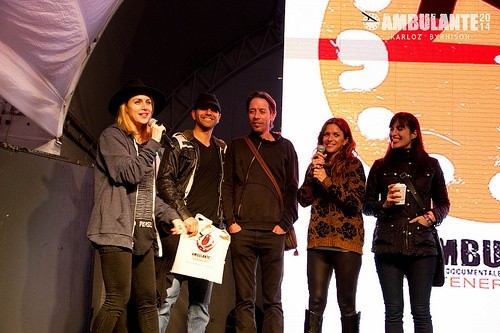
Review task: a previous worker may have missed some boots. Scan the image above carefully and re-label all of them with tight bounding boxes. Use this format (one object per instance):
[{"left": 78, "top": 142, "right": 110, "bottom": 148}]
[
  {"left": 304, "top": 309, "right": 323, "bottom": 333},
  {"left": 341, "top": 311, "right": 360, "bottom": 333}
]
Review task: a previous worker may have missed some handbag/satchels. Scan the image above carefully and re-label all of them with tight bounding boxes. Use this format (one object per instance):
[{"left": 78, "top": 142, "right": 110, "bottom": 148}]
[
  {"left": 283, "top": 226, "right": 297, "bottom": 249},
  {"left": 432, "top": 231, "right": 445, "bottom": 286}
]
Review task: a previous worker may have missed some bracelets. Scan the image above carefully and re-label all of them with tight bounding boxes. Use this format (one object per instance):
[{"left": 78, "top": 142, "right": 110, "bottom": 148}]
[{"left": 423, "top": 214, "right": 433, "bottom": 225}]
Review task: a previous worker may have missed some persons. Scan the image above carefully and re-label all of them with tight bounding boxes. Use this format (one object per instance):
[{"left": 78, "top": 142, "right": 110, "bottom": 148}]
[
  {"left": 297, "top": 118, "right": 366, "bottom": 333},
  {"left": 221, "top": 91, "right": 299, "bottom": 333},
  {"left": 363, "top": 112, "right": 450, "bottom": 333},
  {"left": 86, "top": 78, "right": 187, "bottom": 333},
  {"left": 157, "top": 94, "right": 228, "bottom": 333}
]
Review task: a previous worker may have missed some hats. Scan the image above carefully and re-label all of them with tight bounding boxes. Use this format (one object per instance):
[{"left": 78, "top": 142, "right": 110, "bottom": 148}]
[
  {"left": 195, "top": 93, "right": 220, "bottom": 113},
  {"left": 109, "top": 83, "right": 168, "bottom": 114}
]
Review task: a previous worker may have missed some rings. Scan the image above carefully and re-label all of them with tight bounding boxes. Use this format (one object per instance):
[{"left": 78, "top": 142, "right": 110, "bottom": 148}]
[{"left": 156, "top": 121, "right": 161, "bottom": 126}]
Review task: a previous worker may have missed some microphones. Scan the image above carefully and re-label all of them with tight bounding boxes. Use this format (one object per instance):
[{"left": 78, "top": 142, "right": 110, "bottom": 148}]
[
  {"left": 148, "top": 118, "right": 175, "bottom": 150},
  {"left": 317, "top": 145, "right": 325, "bottom": 168}
]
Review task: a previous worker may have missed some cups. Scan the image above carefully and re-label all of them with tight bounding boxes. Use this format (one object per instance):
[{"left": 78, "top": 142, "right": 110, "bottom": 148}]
[{"left": 393, "top": 183, "right": 406, "bottom": 205}]
